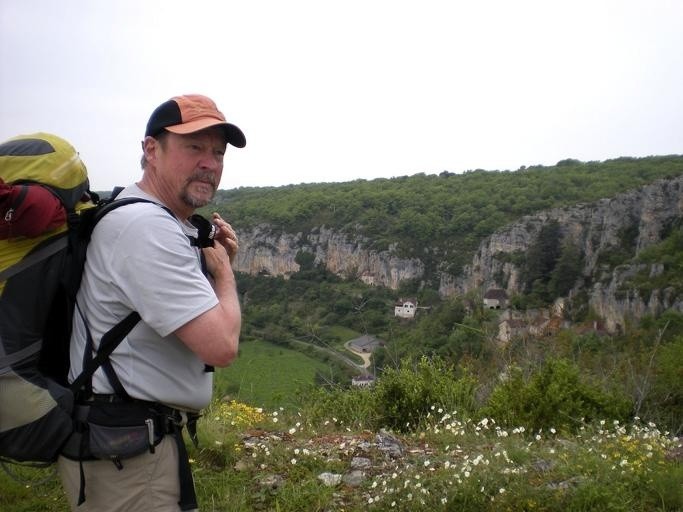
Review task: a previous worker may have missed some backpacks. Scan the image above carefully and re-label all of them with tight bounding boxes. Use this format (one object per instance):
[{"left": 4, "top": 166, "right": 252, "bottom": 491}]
[{"left": 0, "top": 122, "right": 181, "bottom": 475}]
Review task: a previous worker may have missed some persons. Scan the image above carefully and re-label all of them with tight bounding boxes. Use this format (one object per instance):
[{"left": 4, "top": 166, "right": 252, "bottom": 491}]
[{"left": 52, "top": 88, "right": 253, "bottom": 512}]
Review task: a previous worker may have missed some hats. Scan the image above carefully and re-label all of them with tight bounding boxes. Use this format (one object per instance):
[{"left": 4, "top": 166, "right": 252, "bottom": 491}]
[{"left": 144, "top": 94, "right": 249, "bottom": 150}]
